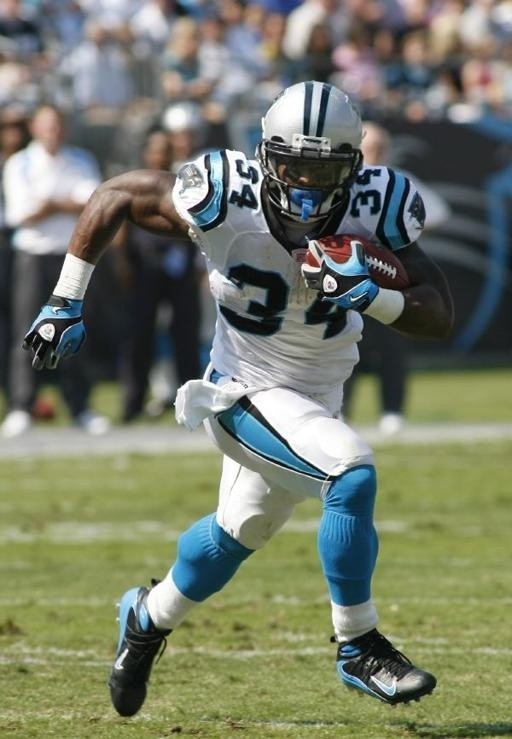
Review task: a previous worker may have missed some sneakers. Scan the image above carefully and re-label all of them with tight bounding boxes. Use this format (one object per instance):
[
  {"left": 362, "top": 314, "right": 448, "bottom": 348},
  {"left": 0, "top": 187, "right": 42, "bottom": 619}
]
[
  {"left": 330, "top": 627, "right": 438, "bottom": 707},
  {"left": 107, "top": 584, "right": 174, "bottom": 718}
]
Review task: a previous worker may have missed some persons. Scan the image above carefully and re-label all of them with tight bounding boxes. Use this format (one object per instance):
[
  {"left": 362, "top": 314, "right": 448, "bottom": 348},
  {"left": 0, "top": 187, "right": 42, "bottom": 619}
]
[{"left": 21, "top": 80, "right": 457, "bottom": 716}]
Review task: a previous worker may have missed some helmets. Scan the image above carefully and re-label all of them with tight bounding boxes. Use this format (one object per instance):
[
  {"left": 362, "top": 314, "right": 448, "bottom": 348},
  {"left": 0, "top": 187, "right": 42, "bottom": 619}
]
[{"left": 254, "top": 78, "right": 364, "bottom": 225}]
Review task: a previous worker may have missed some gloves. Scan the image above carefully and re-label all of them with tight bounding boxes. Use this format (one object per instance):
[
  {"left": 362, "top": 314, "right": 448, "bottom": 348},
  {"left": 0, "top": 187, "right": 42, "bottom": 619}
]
[
  {"left": 299, "top": 240, "right": 408, "bottom": 327},
  {"left": 22, "top": 251, "right": 97, "bottom": 371}
]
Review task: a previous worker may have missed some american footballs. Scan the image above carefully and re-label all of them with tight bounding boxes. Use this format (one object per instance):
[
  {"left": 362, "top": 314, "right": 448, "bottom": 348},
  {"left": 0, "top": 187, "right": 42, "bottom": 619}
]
[{"left": 305, "top": 233, "right": 411, "bottom": 290}]
